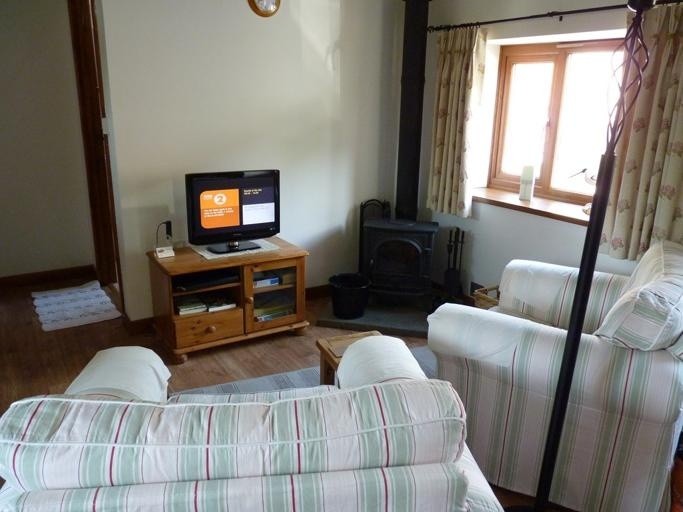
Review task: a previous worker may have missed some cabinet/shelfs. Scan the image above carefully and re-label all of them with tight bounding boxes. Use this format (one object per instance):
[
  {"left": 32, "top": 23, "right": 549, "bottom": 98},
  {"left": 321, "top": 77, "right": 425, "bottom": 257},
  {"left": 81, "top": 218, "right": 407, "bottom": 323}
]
[{"left": 145, "top": 237, "right": 308, "bottom": 363}]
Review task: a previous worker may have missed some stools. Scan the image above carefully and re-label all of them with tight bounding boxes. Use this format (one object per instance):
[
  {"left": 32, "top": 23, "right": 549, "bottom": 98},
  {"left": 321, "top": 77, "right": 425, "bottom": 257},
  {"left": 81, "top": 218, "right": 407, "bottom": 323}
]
[{"left": 316, "top": 331, "right": 384, "bottom": 385}]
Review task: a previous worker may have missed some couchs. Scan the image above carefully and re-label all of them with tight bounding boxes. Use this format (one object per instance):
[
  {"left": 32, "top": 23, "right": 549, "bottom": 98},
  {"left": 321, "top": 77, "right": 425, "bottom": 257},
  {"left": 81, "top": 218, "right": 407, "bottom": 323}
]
[
  {"left": 428, "top": 244, "right": 682, "bottom": 512},
  {"left": 0, "top": 335, "right": 504, "bottom": 512}
]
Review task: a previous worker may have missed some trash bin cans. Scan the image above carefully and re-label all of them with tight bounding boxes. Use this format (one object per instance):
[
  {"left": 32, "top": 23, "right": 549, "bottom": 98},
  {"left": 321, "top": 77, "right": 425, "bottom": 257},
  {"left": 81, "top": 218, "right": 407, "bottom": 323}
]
[{"left": 328, "top": 273, "right": 372, "bottom": 320}]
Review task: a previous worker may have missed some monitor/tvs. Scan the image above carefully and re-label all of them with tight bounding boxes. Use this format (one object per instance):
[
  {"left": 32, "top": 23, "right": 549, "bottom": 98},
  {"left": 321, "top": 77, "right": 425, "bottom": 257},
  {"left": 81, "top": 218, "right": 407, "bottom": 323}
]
[{"left": 185, "top": 169, "right": 280, "bottom": 254}]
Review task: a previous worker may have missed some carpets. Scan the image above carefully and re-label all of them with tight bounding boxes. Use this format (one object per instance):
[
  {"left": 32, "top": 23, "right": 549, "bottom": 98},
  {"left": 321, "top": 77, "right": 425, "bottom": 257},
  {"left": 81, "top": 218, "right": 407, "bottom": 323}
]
[{"left": 31, "top": 280, "right": 122, "bottom": 331}]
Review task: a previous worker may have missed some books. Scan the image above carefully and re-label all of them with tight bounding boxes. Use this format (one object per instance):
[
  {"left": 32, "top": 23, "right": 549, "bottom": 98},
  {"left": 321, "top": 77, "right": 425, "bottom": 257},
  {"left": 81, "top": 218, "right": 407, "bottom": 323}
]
[
  {"left": 175, "top": 291, "right": 237, "bottom": 316},
  {"left": 253, "top": 289, "right": 295, "bottom": 322}
]
